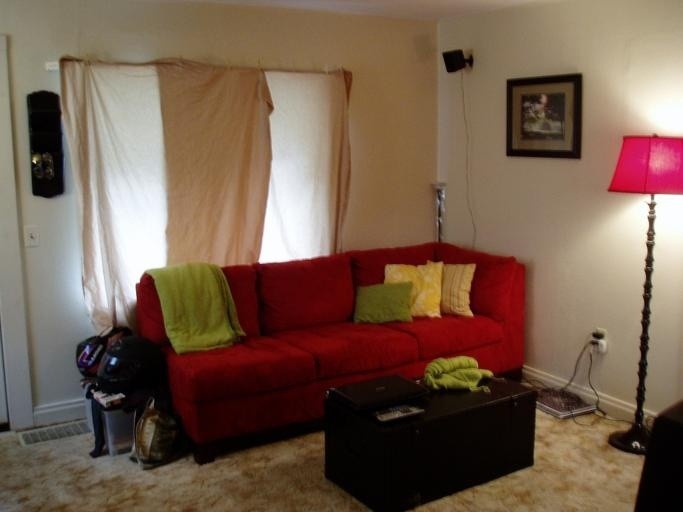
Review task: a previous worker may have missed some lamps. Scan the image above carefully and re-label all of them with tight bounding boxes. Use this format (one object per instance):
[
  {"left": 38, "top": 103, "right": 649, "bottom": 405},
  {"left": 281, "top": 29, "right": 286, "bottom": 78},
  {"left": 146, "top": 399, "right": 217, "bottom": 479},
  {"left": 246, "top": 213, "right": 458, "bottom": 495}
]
[{"left": 606, "top": 134, "right": 683, "bottom": 456}]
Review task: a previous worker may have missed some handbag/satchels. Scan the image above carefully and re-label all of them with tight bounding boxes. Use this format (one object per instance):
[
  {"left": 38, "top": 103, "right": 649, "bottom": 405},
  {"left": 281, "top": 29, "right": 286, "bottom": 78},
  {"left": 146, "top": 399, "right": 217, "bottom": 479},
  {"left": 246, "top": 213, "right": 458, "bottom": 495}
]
[
  {"left": 129, "top": 407, "right": 179, "bottom": 463},
  {"left": 76, "top": 326, "right": 150, "bottom": 392}
]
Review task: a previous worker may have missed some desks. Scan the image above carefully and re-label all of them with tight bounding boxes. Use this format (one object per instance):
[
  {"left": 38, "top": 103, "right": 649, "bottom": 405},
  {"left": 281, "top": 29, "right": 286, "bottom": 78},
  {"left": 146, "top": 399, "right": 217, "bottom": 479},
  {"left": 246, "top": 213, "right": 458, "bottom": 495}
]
[{"left": 324, "top": 373, "right": 539, "bottom": 512}]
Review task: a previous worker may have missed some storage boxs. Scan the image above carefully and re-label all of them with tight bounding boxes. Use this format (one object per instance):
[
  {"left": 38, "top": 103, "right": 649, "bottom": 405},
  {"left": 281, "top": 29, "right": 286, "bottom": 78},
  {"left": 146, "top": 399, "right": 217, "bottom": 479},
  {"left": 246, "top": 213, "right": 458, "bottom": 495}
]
[{"left": 83, "top": 389, "right": 134, "bottom": 456}]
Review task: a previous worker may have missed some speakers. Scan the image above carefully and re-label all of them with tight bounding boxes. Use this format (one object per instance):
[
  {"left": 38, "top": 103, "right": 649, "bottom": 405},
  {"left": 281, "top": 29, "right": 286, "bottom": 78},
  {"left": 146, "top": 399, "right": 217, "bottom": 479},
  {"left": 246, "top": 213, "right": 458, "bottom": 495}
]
[{"left": 441, "top": 47, "right": 467, "bottom": 74}]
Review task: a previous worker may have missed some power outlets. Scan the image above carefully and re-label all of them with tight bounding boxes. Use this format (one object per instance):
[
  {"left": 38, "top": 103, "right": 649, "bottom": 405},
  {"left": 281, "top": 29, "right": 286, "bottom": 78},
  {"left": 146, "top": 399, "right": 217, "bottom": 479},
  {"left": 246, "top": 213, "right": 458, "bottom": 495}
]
[{"left": 592, "top": 328, "right": 607, "bottom": 353}]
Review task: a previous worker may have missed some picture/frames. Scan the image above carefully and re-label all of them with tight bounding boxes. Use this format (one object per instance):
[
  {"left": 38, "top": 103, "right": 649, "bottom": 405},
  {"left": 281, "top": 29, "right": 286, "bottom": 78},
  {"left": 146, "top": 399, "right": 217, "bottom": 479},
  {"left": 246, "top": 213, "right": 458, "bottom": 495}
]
[{"left": 506, "top": 73, "right": 582, "bottom": 159}]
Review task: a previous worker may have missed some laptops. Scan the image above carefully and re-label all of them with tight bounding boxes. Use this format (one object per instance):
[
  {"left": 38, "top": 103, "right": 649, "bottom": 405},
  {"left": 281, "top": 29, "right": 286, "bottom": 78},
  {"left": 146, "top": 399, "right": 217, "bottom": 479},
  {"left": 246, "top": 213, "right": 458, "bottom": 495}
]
[{"left": 326, "top": 374, "right": 432, "bottom": 413}]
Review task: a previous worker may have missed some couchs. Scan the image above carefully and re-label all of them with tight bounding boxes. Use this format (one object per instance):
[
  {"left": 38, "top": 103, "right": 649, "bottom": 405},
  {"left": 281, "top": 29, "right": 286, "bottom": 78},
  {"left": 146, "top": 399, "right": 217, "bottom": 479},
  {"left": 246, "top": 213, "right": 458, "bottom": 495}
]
[{"left": 135, "top": 241, "right": 526, "bottom": 465}]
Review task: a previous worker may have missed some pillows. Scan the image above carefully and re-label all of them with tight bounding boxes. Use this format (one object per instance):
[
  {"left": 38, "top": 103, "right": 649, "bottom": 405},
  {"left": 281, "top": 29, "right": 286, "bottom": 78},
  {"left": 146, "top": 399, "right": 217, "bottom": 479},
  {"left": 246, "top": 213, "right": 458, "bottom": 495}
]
[{"left": 353, "top": 260, "right": 477, "bottom": 323}]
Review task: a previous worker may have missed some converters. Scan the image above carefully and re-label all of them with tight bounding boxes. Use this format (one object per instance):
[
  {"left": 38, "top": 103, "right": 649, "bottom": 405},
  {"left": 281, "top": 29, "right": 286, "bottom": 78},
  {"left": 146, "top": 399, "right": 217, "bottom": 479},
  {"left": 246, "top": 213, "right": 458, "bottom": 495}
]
[{"left": 592, "top": 332, "right": 606, "bottom": 353}]
